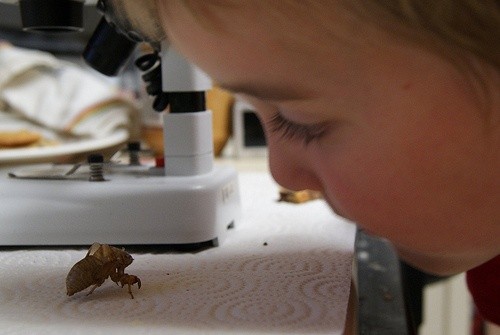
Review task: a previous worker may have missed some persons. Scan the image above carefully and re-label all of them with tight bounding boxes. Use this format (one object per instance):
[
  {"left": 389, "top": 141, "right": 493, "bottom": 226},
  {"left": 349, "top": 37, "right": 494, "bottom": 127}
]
[{"left": 120, "top": 0, "right": 500, "bottom": 328}]
